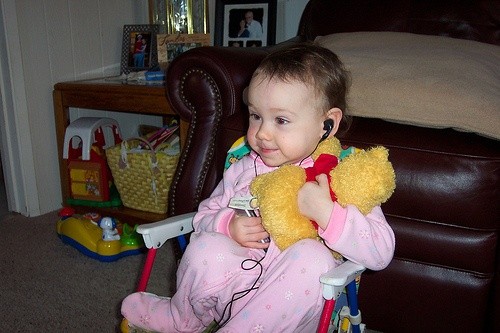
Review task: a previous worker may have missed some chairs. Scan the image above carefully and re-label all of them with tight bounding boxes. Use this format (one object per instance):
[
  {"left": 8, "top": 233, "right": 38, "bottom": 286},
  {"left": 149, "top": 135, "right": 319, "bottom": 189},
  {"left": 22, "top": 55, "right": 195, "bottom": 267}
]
[{"left": 118, "top": 211, "right": 367, "bottom": 333}]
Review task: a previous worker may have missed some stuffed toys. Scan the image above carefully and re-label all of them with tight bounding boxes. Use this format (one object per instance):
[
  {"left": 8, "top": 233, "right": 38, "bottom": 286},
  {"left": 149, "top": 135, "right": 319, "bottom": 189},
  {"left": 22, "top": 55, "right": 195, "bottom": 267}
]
[{"left": 250, "top": 138, "right": 396, "bottom": 259}]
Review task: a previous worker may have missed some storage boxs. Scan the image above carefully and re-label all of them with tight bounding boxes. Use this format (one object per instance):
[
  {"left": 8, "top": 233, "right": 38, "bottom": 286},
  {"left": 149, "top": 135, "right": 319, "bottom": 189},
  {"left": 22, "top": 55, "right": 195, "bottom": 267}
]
[
  {"left": 144, "top": 70, "right": 166, "bottom": 81},
  {"left": 67, "top": 158, "right": 110, "bottom": 203}
]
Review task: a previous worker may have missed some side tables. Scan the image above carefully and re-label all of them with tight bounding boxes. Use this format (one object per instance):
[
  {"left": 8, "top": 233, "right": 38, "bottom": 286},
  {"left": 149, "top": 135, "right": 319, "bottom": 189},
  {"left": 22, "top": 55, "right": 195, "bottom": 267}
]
[{"left": 52, "top": 74, "right": 193, "bottom": 226}]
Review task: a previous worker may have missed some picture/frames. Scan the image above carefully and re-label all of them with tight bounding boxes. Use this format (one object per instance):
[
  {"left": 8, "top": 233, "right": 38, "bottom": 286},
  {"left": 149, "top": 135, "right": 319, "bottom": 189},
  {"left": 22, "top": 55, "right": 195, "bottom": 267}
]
[
  {"left": 119, "top": 24, "right": 159, "bottom": 74},
  {"left": 156, "top": 34, "right": 210, "bottom": 62},
  {"left": 213, "top": 0, "right": 278, "bottom": 48}
]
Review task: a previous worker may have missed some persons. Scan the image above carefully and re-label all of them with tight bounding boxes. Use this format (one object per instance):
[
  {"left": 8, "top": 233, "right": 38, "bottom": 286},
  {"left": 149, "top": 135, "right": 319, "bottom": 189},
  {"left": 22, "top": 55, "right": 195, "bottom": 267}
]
[
  {"left": 233, "top": 11, "right": 263, "bottom": 38},
  {"left": 172, "top": 44, "right": 190, "bottom": 58},
  {"left": 134, "top": 34, "right": 146, "bottom": 67},
  {"left": 232, "top": 42, "right": 240, "bottom": 48},
  {"left": 249, "top": 43, "right": 256, "bottom": 48},
  {"left": 120, "top": 44, "right": 396, "bottom": 333}
]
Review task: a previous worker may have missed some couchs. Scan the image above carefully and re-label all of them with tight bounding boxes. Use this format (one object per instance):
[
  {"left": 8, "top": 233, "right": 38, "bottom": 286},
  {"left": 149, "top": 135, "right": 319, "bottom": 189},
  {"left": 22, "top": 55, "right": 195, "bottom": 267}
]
[{"left": 164, "top": 0, "right": 500, "bottom": 333}]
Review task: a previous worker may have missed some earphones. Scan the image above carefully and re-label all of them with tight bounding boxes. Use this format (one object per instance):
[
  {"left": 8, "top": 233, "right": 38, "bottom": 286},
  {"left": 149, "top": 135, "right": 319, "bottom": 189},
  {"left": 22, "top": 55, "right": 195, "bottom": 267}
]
[{"left": 322, "top": 119, "right": 334, "bottom": 139}]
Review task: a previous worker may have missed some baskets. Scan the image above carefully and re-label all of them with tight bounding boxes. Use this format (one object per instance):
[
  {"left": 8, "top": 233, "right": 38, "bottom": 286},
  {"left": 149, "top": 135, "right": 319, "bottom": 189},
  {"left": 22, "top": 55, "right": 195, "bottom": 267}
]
[{"left": 105, "top": 131, "right": 181, "bottom": 214}]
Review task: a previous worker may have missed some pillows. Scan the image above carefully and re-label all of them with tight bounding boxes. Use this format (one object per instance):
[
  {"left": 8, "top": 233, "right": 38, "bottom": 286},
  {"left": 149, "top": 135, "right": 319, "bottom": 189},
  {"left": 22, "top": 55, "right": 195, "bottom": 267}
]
[{"left": 313, "top": 31, "right": 500, "bottom": 143}]
